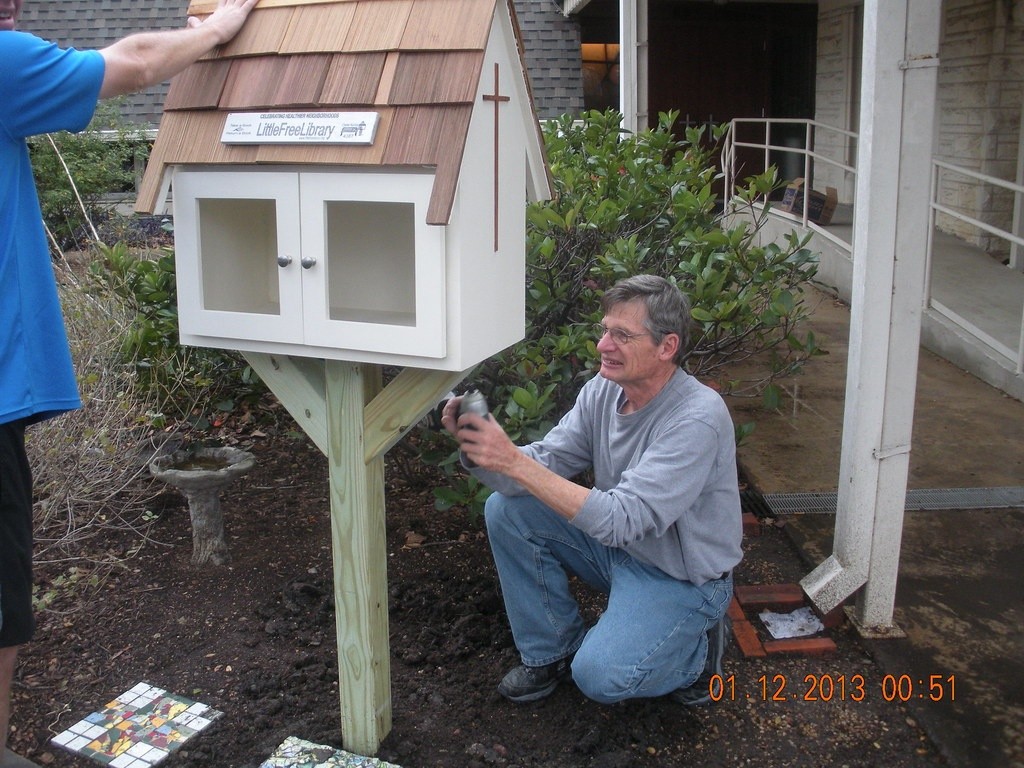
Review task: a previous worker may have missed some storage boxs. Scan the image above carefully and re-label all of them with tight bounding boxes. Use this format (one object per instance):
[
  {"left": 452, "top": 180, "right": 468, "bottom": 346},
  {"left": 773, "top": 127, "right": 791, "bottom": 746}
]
[{"left": 782, "top": 178, "right": 838, "bottom": 225}]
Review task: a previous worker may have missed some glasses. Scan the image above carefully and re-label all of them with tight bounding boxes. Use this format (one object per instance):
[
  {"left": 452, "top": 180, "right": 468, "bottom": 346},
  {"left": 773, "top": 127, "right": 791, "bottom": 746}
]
[{"left": 592, "top": 323, "right": 651, "bottom": 346}]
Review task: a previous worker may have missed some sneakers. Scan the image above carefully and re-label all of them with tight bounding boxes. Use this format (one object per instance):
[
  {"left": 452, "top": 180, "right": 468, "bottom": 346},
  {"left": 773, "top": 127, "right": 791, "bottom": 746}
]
[
  {"left": 498, "top": 650, "right": 576, "bottom": 701},
  {"left": 674, "top": 616, "right": 723, "bottom": 706}
]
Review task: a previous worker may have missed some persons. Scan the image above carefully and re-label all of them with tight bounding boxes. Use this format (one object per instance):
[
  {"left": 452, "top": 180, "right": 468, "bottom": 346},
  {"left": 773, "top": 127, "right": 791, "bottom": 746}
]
[
  {"left": 0, "top": 0, "right": 262, "bottom": 754},
  {"left": 442, "top": 273, "right": 742, "bottom": 705}
]
